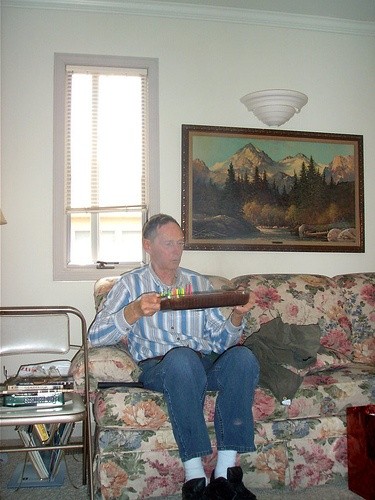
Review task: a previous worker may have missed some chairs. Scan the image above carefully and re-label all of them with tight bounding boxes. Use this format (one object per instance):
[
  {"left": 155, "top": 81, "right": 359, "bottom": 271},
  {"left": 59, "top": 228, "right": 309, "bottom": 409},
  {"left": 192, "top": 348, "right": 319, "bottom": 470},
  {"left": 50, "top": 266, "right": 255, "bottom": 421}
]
[{"left": 0, "top": 307, "right": 94, "bottom": 500}]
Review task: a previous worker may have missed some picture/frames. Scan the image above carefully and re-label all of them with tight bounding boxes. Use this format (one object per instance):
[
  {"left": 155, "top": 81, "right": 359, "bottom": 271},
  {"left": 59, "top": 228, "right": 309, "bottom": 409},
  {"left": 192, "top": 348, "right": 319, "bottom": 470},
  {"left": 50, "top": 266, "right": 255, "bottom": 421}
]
[{"left": 182, "top": 124, "right": 365, "bottom": 253}]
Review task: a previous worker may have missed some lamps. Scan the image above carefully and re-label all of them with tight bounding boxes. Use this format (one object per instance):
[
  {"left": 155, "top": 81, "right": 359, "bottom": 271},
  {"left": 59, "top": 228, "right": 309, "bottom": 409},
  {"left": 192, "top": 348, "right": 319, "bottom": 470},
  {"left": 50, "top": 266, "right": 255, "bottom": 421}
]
[{"left": 241, "top": 89, "right": 308, "bottom": 126}]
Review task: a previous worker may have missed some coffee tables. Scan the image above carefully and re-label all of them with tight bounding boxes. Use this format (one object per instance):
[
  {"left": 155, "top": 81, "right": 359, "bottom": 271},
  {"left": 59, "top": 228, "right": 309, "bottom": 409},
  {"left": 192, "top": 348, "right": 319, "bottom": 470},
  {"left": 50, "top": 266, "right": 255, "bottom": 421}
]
[{"left": 346, "top": 406, "right": 375, "bottom": 500}]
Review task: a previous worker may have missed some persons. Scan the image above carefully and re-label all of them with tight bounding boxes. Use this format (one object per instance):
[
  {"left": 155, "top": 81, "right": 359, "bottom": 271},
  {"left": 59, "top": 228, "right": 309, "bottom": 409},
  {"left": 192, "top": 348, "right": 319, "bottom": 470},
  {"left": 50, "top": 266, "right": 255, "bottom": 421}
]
[{"left": 88, "top": 214, "right": 260, "bottom": 500}]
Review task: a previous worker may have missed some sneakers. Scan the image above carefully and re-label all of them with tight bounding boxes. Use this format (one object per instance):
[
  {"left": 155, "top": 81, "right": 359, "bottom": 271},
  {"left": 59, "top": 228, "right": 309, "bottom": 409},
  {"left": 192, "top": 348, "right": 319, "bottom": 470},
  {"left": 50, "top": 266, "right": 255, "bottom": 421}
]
[
  {"left": 204, "top": 466, "right": 257, "bottom": 500},
  {"left": 181, "top": 477, "right": 206, "bottom": 500}
]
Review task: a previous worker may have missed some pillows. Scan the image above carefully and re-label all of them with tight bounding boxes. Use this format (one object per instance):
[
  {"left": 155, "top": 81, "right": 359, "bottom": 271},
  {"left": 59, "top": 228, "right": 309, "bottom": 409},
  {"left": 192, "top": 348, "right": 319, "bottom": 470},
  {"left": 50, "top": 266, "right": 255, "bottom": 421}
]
[{"left": 282, "top": 346, "right": 352, "bottom": 378}]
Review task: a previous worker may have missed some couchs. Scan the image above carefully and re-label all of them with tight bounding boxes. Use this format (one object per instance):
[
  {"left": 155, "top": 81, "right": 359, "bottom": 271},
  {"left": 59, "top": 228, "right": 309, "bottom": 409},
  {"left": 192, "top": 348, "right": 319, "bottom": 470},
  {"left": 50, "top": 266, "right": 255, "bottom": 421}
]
[{"left": 70, "top": 272, "right": 375, "bottom": 500}]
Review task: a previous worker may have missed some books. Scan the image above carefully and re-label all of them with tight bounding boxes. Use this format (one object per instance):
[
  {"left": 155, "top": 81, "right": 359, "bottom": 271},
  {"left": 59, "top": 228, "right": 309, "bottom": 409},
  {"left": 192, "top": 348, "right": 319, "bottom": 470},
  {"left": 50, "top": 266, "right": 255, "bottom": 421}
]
[{"left": 17, "top": 422, "right": 73, "bottom": 482}]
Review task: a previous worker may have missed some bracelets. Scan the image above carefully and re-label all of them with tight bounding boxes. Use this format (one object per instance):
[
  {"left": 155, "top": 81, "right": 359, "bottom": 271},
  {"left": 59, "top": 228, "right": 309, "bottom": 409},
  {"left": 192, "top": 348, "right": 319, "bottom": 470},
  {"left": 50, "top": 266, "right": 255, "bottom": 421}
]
[{"left": 234, "top": 310, "right": 247, "bottom": 316}]
